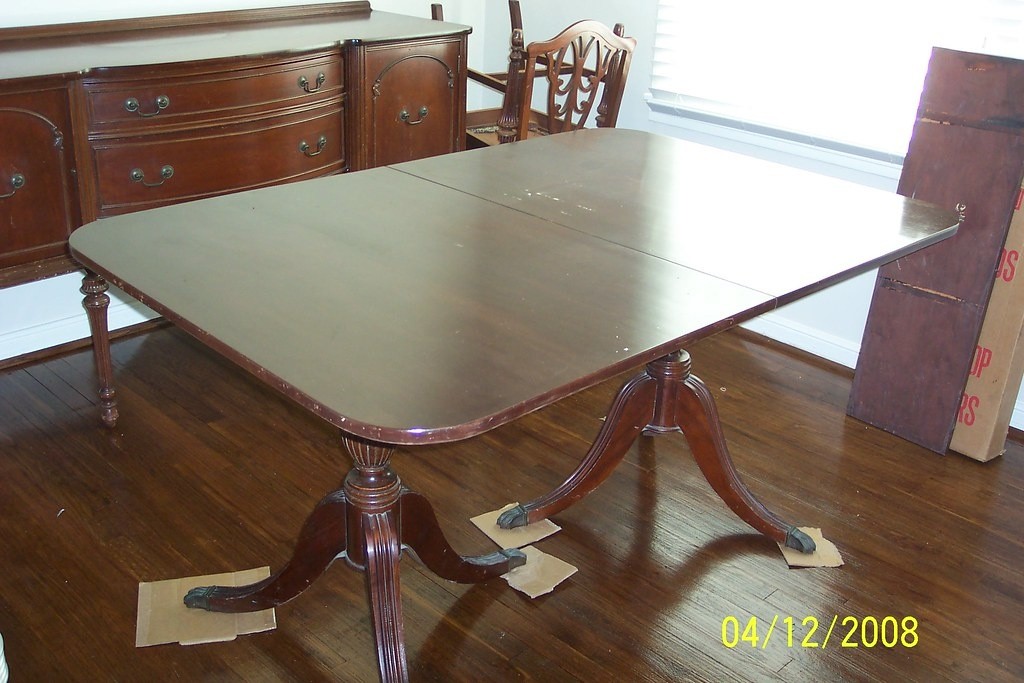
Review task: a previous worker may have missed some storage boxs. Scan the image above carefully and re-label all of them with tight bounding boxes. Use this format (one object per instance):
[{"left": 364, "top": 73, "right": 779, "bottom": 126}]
[{"left": 948, "top": 176, "right": 1024, "bottom": 464}]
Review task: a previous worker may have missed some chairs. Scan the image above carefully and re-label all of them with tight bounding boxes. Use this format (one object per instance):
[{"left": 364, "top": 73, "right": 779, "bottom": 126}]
[{"left": 466, "top": 20, "right": 638, "bottom": 147}]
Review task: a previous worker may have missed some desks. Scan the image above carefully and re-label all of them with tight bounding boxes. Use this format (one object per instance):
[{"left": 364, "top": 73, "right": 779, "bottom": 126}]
[{"left": 69, "top": 125, "right": 962, "bottom": 683}]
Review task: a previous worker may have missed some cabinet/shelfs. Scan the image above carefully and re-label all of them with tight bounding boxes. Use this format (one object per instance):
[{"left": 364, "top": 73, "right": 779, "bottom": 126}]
[
  {"left": 0, "top": 0, "right": 474, "bottom": 430},
  {"left": 845, "top": 44, "right": 1024, "bottom": 456}
]
